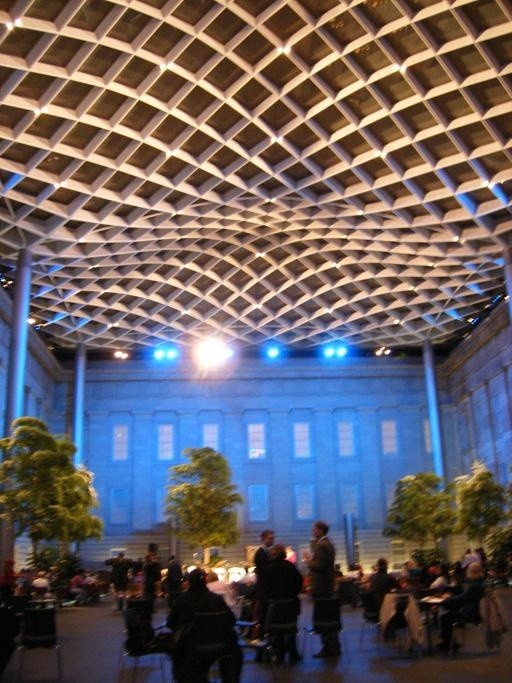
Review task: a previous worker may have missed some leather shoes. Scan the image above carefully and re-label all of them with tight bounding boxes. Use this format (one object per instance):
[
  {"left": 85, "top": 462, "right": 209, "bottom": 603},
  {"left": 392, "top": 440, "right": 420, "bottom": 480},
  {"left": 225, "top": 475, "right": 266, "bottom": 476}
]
[{"left": 314, "top": 643, "right": 342, "bottom": 658}]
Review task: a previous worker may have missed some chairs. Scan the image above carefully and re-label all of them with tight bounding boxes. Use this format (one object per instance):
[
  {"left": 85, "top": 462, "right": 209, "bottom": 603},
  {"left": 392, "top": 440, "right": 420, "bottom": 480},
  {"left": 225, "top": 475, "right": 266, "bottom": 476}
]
[
  {"left": 126, "top": 596, "right": 242, "bottom": 683},
  {"left": 361, "top": 577, "right": 512, "bottom": 655},
  {"left": 233, "top": 589, "right": 344, "bottom": 659},
  {"left": 17, "top": 609, "right": 65, "bottom": 681}
]
